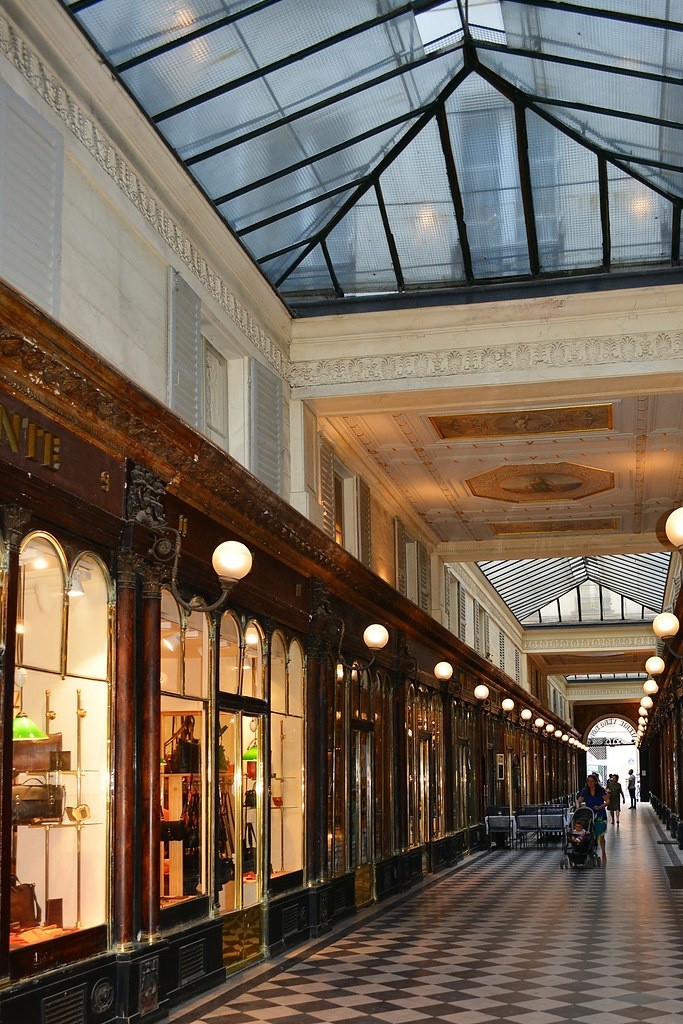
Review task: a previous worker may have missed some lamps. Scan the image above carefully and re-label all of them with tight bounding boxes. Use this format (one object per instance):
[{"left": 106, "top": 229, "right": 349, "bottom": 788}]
[
  {"left": 665, "top": 506, "right": 683, "bottom": 556},
  {"left": 66, "top": 565, "right": 85, "bottom": 597},
  {"left": 162, "top": 622, "right": 252, "bottom": 669},
  {"left": 486, "top": 696, "right": 515, "bottom": 722},
  {"left": 527, "top": 718, "right": 588, "bottom": 753},
  {"left": 126, "top": 462, "right": 252, "bottom": 613},
  {"left": 311, "top": 576, "right": 389, "bottom": 671},
  {"left": 452, "top": 678, "right": 489, "bottom": 712},
  {"left": 395, "top": 629, "right": 453, "bottom": 695},
  {"left": 635, "top": 613, "right": 683, "bottom": 749},
  {"left": 510, "top": 709, "right": 532, "bottom": 729}
]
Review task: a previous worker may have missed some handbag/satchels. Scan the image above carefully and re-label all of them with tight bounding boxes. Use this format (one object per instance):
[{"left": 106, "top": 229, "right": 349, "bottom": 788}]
[
  {"left": 160, "top": 715, "right": 273, "bottom": 909},
  {"left": 11, "top": 873, "right": 41, "bottom": 929},
  {"left": 12, "top": 778, "right": 63, "bottom": 822}
]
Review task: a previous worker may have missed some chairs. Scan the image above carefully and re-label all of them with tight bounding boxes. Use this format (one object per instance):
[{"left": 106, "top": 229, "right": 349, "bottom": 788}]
[
  {"left": 487, "top": 804, "right": 570, "bottom": 852},
  {"left": 449, "top": 214, "right": 566, "bottom": 282}
]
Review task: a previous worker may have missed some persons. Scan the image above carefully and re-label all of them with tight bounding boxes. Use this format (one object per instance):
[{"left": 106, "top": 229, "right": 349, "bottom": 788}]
[
  {"left": 576, "top": 775, "right": 610, "bottom": 861},
  {"left": 608, "top": 774, "right": 626, "bottom": 824},
  {"left": 627, "top": 769, "right": 636, "bottom": 809},
  {"left": 572, "top": 822, "right": 586, "bottom": 842}
]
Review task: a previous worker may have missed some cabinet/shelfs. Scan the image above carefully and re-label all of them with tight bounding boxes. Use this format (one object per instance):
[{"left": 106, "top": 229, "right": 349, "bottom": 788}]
[
  {"left": 11, "top": 688, "right": 103, "bottom": 949},
  {"left": 240, "top": 776, "right": 298, "bottom": 884}
]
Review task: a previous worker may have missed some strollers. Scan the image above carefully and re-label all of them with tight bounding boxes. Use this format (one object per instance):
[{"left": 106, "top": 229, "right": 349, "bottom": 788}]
[{"left": 560, "top": 805, "right": 600, "bottom": 871}]
